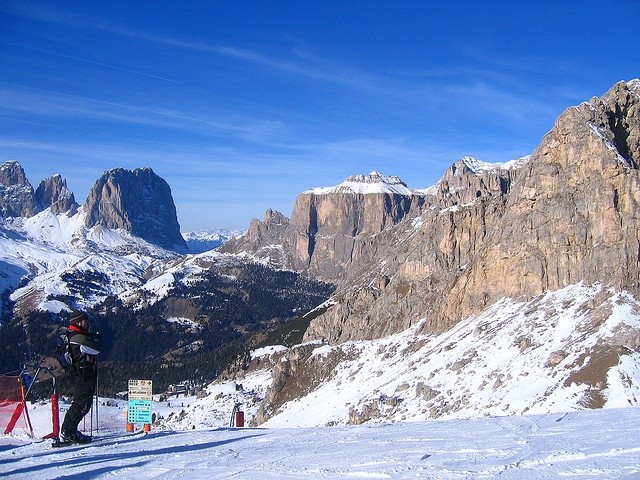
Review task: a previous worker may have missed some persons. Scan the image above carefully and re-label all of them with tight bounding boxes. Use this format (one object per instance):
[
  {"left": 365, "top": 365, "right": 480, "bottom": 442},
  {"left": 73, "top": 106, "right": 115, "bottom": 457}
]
[{"left": 55, "top": 310, "right": 101, "bottom": 444}]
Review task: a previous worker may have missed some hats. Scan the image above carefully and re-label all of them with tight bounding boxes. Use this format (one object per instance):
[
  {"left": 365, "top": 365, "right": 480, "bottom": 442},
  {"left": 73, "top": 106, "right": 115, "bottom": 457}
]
[{"left": 69, "top": 309, "right": 87, "bottom": 324}]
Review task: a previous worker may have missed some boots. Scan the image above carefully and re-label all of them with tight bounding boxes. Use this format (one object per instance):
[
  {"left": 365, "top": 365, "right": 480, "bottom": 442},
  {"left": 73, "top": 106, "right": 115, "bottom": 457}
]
[
  {"left": 61, "top": 430, "right": 80, "bottom": 444},
  {"left": 76, "top": 431, "right": 94, "bottom": 442}
]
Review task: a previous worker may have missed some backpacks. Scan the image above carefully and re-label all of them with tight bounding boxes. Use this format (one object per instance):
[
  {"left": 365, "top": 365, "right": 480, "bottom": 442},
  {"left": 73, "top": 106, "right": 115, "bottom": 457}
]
[{"left": 54, "top": 332, "right": 96, "bottom": 377}]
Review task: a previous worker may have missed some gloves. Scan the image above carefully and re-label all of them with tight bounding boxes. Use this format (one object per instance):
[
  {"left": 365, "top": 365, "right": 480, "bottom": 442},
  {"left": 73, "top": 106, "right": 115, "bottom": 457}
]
[{"left": 94, "top": 331, "right": 100, "bottom": 340}]
